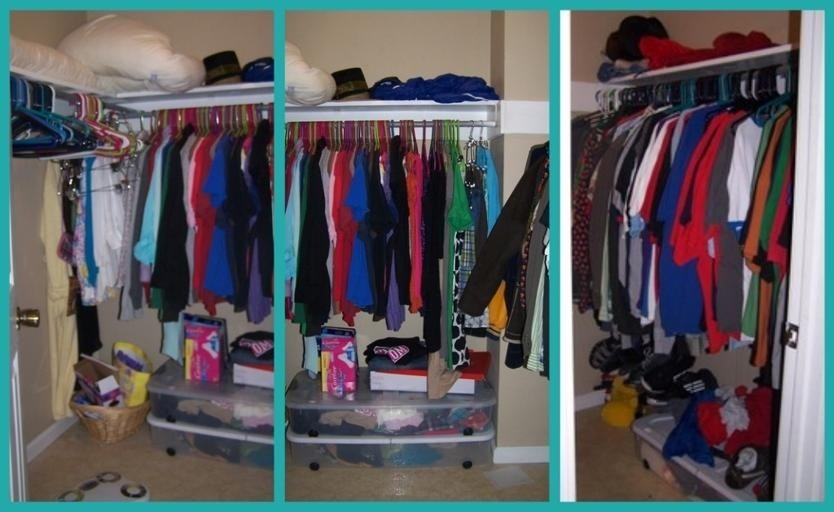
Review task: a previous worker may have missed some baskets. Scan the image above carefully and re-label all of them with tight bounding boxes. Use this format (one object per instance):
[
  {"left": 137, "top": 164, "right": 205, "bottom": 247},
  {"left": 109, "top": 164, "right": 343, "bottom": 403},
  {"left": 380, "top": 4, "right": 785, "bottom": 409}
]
[{"left": 69, "top": 396, "right": 150, "bottom": 445}]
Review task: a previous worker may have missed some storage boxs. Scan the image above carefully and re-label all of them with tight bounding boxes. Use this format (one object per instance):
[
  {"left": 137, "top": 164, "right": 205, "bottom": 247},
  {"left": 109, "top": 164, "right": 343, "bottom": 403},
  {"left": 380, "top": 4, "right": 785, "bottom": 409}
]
[
  {"left": 631, "top": 407, "right": 767, "bottom": 502},
  {"left": 143, "top": 353, "right": 501, "bottom": 468}
]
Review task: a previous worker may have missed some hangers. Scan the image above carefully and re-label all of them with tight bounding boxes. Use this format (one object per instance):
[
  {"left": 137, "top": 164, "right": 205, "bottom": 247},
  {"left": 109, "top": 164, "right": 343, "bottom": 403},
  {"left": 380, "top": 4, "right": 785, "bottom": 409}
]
[
  {"left": 9, "top": 73, "right": 489, "bottom": 174},
  {"left": 585, "top": 64, "right": 795, "bottom": 124}
]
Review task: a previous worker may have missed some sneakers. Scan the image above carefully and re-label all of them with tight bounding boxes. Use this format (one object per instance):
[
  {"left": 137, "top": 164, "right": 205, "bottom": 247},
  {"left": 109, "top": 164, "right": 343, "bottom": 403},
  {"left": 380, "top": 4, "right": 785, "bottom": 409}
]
[
  {"left": 589, "top": 336, "right": 720, "bottom": 419},
  {"left": 723, "top": 445, "right": 771, "bottom": 489}
]
